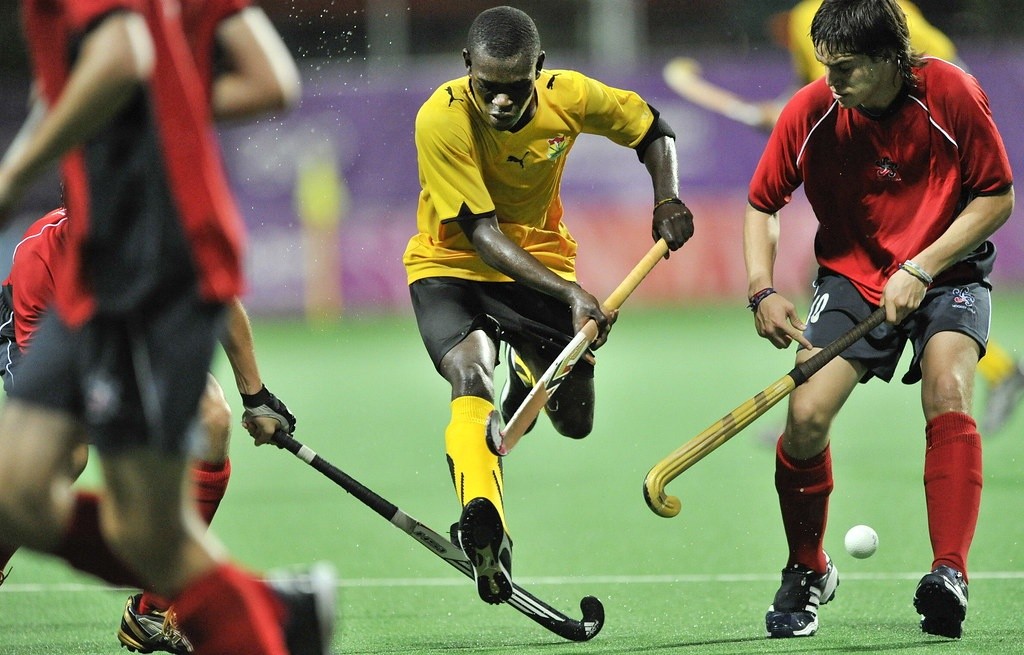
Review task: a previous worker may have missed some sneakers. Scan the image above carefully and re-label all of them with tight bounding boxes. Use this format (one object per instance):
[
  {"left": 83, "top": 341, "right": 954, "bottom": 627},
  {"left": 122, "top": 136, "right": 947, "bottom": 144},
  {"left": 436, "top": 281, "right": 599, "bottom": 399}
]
[
  {"left": 499, "top": 341, "right": 541, "bottom": 436},
  {"left": 117, "top": 592, "right": 195, "bottom": 655},
  {"left": 457, "top": 497, "right": 514, "bottom": 605},
  {"left": 765, "top": 547, "right": 840, "bottom": 637},
  {"left": 913, "top": 564, "right": 969, "bottom": 639},
  {"left": 984, "top": 368, "right": 1024, "bottom": 432},
  {"left": 262, "top": 562, "right": 336, "bottom": 655}
]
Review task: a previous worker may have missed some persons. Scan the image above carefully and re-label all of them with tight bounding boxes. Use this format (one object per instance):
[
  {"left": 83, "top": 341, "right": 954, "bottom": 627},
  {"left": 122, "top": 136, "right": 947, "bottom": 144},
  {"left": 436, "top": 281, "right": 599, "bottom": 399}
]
[
  {"left": 0, "top": 0, "right": 339, "bottom": 655},
  {"left": 742, "top": 1, "right": 1018, "bottom": 639},
  {"left": 400, "top": 5, "right": 696, "bottom": 607},
  {"left": 0, "top": 172, "right": 300, "bottom": 655}
]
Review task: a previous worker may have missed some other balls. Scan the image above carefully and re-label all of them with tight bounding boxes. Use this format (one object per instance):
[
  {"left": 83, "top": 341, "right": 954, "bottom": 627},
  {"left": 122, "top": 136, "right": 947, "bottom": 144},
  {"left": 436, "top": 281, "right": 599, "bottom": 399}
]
[{"left": 845, "top": 525, "right": 880, "bottom": 559}]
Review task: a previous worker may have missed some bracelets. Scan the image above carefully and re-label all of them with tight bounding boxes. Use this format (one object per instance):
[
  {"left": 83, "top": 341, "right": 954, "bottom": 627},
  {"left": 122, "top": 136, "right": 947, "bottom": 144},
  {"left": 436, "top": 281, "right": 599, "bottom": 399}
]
[
  {"left": 900, "top": 259, "right": 934, "bottom": 287},
  {"left": 653, "top": 197, "right": 686, "bottom": 211},
  {"left": 746, "top": 287, "right": 777, "bottom": 312}
]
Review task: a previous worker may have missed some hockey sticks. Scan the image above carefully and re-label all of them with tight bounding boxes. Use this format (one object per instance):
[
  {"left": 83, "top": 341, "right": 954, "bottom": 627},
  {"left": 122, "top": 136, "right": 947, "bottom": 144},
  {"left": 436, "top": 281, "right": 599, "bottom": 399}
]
[{"left": 242, "top": 231, "right": 998, "bottom": 639}]
